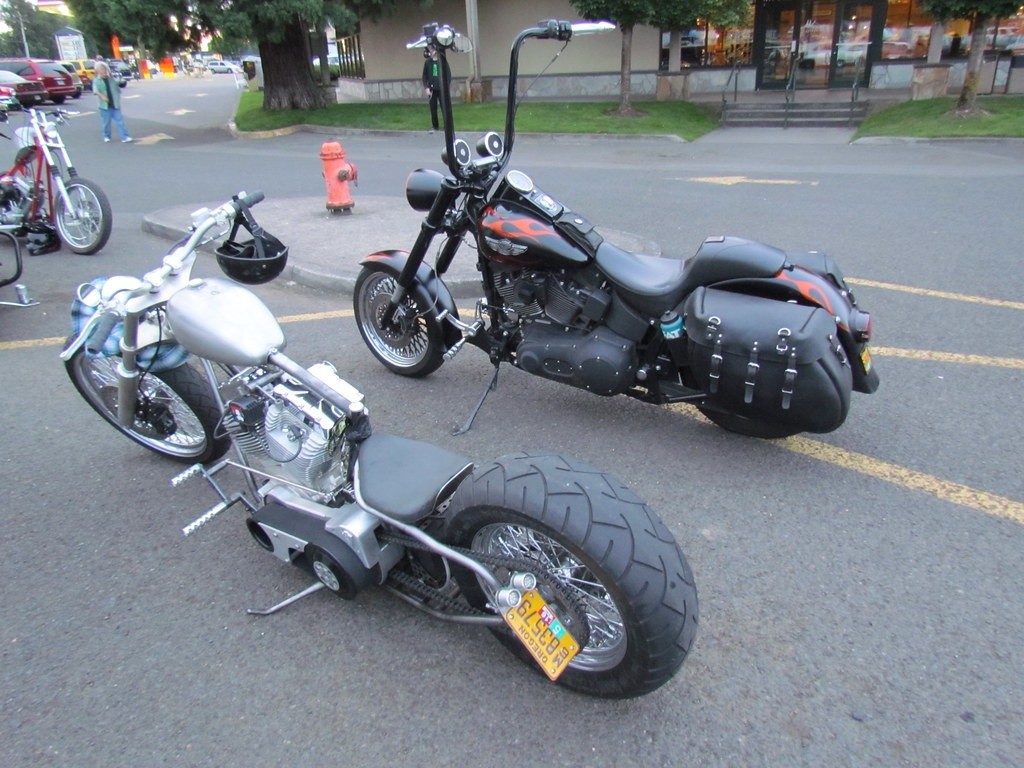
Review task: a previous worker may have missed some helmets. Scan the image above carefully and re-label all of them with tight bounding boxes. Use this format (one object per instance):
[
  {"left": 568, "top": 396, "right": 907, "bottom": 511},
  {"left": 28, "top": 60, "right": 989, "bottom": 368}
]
[{"left": 215, "top": 239, "right": 289, "bottom": 285}]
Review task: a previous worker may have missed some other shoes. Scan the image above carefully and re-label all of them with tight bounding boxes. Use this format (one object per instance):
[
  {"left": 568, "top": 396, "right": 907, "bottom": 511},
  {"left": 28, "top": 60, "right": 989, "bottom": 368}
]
[
  {"left": 104, "top": 138, "right": 110, "bottom": 143},
  {"left": 121, "top": 136, "right": 133, "bottom": 143}
]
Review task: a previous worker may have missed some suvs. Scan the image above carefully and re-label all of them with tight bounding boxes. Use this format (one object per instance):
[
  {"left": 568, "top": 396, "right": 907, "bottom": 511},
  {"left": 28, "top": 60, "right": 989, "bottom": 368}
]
[
  {"left": 102, "top": 58, "right": 135, "bottom": 88},
  {"left": 0, "top": 56, "right": 77, "bottom": 104},
  {"left": 64, "top": 59, "right": 98, "bottom": 91}
]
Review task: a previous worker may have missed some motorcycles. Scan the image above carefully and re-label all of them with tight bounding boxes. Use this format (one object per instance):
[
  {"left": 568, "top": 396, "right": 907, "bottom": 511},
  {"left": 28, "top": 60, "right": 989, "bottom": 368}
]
[
  {"left": 58, "top": 186, "right": 700, "bottom": 701},
  {"left": 0, "top": 98, "right": 113, "bottom": 288},
  {"left": 351, "top": 20, "right": 881, "bottom": 441}
]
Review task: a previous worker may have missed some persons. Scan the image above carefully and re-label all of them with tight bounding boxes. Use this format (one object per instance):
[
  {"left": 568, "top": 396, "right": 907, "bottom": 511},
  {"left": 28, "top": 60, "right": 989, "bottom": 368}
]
[
  {"left": 422, "top": 47, "right": 451, "bottom": 133},
  {"left": 92, "top": 61, "right": 132, "bottom": 143},
  {"left": 96, "top": 54, "right": 103, "bottom": 61}
]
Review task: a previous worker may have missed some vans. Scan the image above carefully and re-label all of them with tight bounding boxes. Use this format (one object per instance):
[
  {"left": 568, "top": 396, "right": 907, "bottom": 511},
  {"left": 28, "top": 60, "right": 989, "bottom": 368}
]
[{"left": 208, "top": 60, "right": 235, "bottom": 74}]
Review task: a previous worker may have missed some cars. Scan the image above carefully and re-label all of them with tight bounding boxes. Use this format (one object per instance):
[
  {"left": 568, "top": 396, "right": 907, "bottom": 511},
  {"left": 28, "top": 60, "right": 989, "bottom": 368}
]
[
  {"left": 55, "top": 59, "right": 84, "bottom": 99},
  {"left": 660, "top": 13, "right": 1023, "bottom": 66},
  {"left": 0, "top": 86, "right": 17, "bottom": 114},
  {"left": 0, "top": 70, "right": 50, "bottom": 108}
]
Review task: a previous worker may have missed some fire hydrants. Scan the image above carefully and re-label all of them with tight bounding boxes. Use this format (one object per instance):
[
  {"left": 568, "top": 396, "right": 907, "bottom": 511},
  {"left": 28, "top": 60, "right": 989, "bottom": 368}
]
[{"left": 319, "top": 141, "right": 358, "bottom": 215}]
